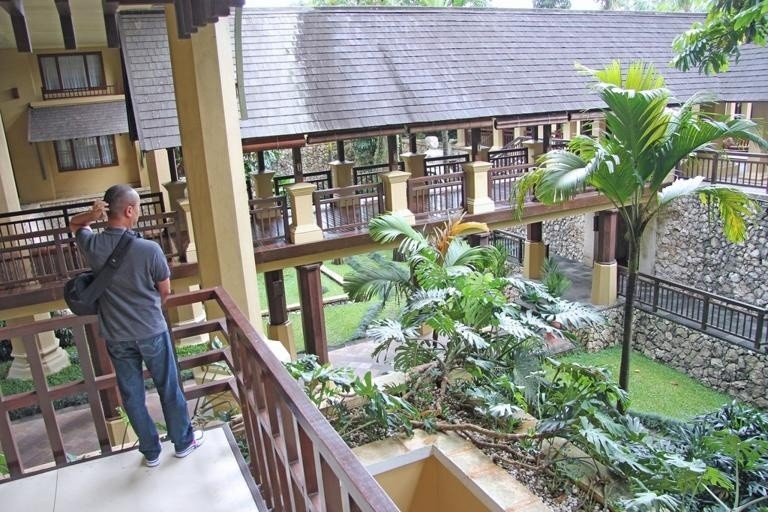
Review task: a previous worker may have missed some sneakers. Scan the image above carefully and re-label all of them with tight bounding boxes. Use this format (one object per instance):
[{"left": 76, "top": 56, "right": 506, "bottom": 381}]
[
  {"left": 173, "top": 429, "right": 204, "bottom": 457},
  {"left": 144, "top": 441, "right": 163, "bottom": 466}
]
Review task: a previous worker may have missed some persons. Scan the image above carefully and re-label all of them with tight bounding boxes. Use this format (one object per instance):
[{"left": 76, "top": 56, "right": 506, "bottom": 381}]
[{"left": 67, "top": 182, "right": 207, "bottom": 469}]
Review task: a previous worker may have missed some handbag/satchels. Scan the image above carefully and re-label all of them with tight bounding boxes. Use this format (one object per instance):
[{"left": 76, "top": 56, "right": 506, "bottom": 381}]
[{"left": 64, "top": 272, "right": 100, "bottom": 315}]
